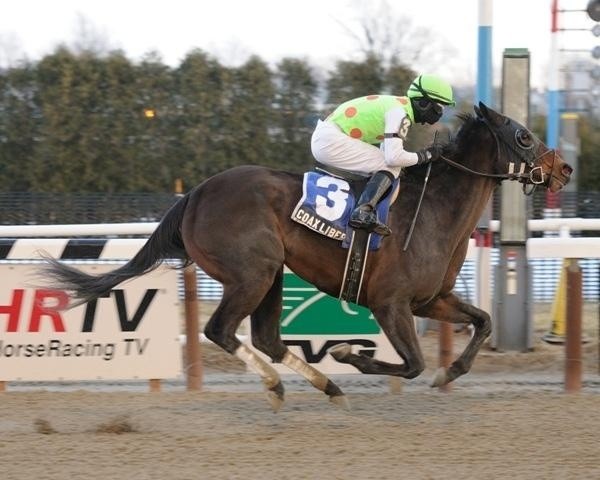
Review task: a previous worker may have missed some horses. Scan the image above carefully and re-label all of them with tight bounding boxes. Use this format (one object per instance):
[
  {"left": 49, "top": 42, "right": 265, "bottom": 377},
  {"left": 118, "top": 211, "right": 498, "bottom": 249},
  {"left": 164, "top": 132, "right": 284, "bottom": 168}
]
[{"left": 21, "top": 99, "right": 574, "bottom": 416}]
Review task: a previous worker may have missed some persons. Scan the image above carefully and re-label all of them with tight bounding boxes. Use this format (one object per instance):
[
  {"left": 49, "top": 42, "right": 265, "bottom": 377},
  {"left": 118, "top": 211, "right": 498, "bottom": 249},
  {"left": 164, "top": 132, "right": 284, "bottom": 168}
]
[{"left": 309, "top": 70, "right": 456, "bottom": 237}]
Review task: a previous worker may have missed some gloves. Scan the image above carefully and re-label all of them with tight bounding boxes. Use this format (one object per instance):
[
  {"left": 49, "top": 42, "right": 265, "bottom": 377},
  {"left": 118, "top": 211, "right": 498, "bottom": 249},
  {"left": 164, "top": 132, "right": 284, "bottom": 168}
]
[{"left": 418, "top": 145, "right": 443, "bottom": 165}]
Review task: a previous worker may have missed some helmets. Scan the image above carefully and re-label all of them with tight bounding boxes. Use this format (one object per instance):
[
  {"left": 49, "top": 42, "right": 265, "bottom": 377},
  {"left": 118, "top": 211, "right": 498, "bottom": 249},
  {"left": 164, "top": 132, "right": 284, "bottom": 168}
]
[{"left": 407, "top": 75, "right": 455, "bottom": 106}]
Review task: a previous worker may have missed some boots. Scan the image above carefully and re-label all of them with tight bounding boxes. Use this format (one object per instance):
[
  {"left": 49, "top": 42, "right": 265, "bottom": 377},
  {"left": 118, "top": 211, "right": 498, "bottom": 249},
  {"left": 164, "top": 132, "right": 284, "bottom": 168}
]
[{"left": 349, "top": 170, "right": 395, "bottom": 236}]
